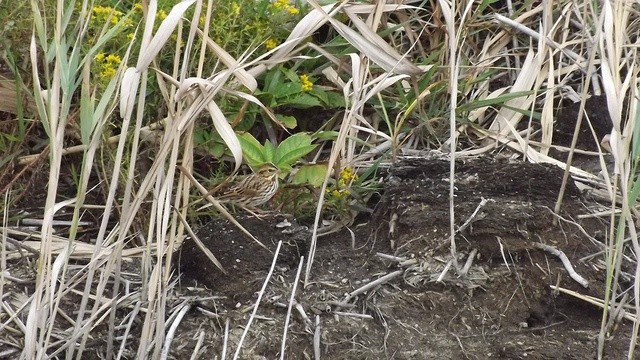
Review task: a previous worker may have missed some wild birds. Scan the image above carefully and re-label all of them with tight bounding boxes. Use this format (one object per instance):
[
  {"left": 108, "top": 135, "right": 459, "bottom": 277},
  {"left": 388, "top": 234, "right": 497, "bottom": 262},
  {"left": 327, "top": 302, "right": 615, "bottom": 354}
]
[{"left": 201, "top": 162, "right": 282, "bottom": 221}]
